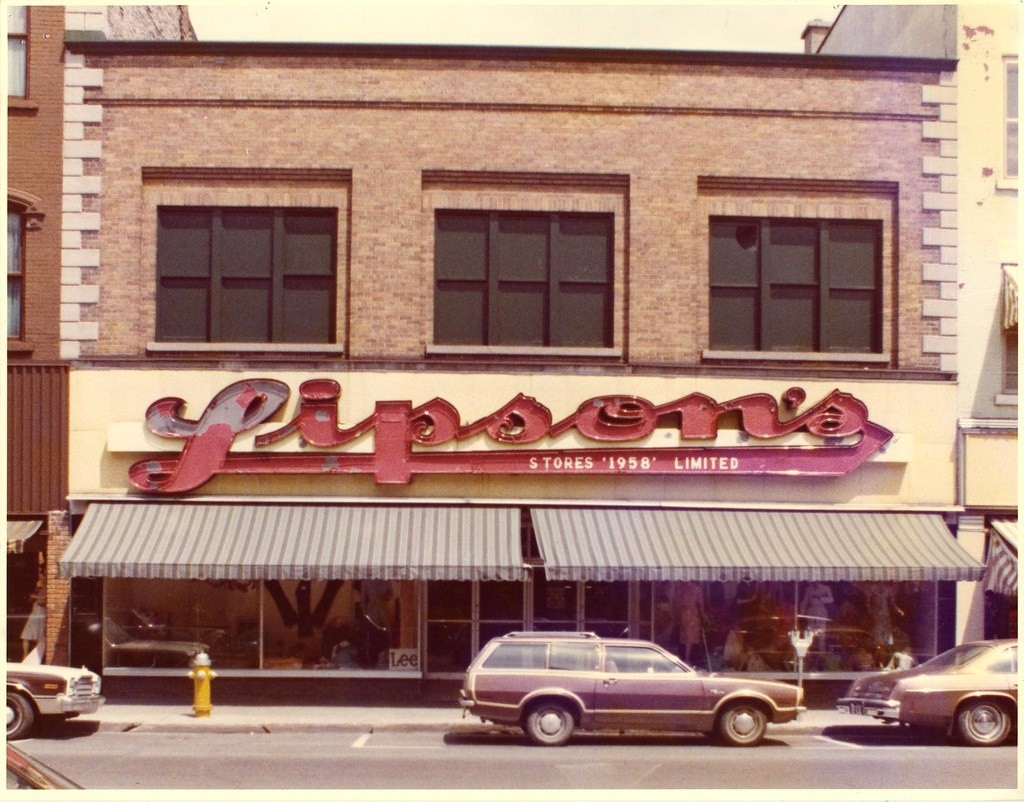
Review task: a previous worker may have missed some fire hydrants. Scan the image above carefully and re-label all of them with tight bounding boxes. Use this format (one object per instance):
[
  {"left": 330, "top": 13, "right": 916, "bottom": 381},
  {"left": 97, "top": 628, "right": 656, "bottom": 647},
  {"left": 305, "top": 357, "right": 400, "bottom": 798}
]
[{"left": 188, "top": 651, "right": 218, "bottom": 717}]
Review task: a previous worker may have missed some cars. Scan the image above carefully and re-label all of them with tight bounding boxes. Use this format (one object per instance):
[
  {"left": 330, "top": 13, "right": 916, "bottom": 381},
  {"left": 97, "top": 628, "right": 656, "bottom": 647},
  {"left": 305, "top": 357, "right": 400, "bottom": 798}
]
[
  {"left": 702, "top": 613, "right": 887, "bottom": 676},
  {"left": 838, "top": 637, "right": 1019, "bottom": 747},
  {"left": 6, "top": 661, "right": 102, "bottom": 739},
  {"left": 71, "top": 617, "right": 471, "bottom": 680}
]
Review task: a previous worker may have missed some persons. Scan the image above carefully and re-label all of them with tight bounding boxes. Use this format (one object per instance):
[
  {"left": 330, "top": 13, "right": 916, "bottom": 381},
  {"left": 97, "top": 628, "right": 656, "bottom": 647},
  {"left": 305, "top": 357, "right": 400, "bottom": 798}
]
[
  {"left": 798, "top": 581, "right": 834, "bottom": 662},
  {"left": 730, "top": 576, "right": 773, "bottom": 665},
  {"left": 20, "top": 562, "right": 48, "bottom": 644},
  {"left": 667, "top": 577, "right": 710, "bottom": 665},
  {"left": 862, "top": 579, "right": 904, "bottom": 670}
]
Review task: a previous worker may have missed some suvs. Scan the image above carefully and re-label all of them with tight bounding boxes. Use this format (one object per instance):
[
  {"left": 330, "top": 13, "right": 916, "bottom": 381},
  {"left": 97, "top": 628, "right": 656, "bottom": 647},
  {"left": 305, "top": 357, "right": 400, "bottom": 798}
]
[{"left": 459, "top": 629, "right": 808, "bottom": 746}]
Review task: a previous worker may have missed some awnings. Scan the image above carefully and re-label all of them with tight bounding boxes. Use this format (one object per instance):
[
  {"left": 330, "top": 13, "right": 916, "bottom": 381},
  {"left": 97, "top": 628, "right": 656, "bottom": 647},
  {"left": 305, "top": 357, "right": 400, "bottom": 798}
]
[
  {"left": 524, "top": 506, "right": 987, "bottom": 582},
  {"left": 55, "top": 500, "right": 530, "bottom": 585},
  {"left": 6, "top": 515, "right": 46, "bottom": 556},
  {"left": 977, "top": 512, "right": 1019, "bottom": 596}
]
